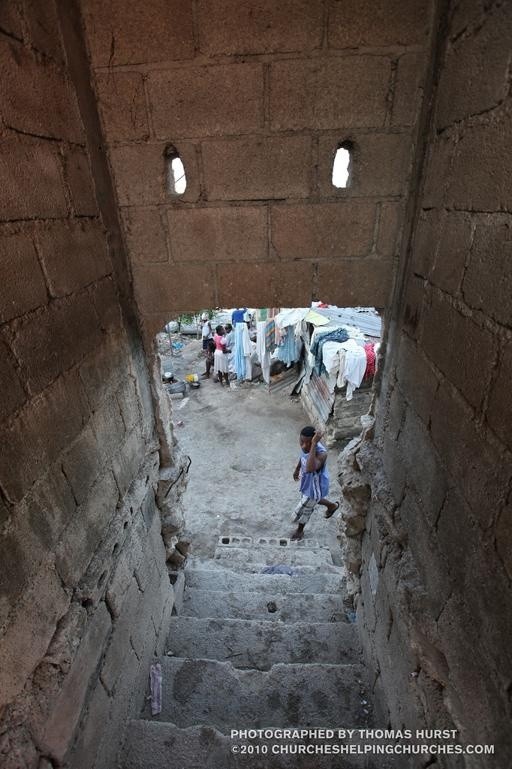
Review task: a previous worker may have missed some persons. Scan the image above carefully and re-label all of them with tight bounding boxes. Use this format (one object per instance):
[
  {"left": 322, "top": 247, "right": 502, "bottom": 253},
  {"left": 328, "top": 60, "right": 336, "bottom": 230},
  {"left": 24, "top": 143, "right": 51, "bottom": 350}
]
[
  {"left": 290, "top": 425, "right": 339, "bottom": 541},
  {"left": 231, "top": 307, "right": 248, "bottom": 328},
  {"left": 199, "top": 341, "right": 217, "bottom": 381},
  {"left": 199, "top": 311, "right": 213, "bottom": 357},
  {"left": 224, "top": 323, "right": 235, "bottom": 348},
  {"left": 213, "top": 324, "right": 232, "bottom": 385}
]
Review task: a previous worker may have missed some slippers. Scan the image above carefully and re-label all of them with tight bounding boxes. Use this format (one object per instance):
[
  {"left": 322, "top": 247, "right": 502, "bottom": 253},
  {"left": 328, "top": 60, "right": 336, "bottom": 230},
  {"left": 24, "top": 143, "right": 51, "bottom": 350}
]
[
  {"left": 323, "top": 501, "right": 340, "bottom": 519},
  {"left": 291, "top": 532, "right": 304, "bottom": 541}
]
